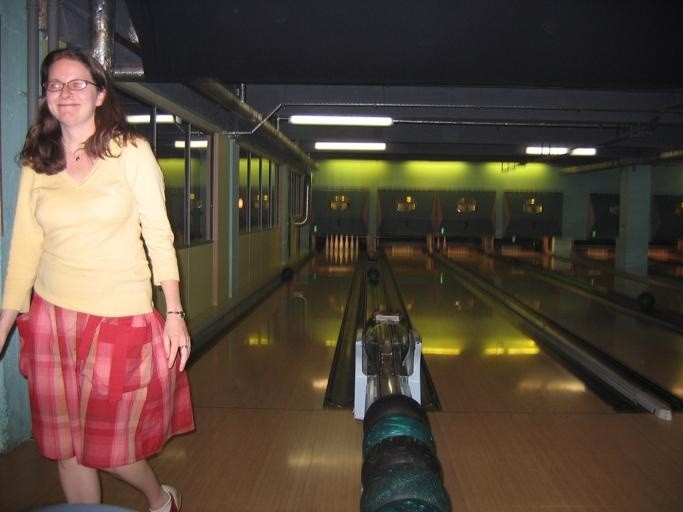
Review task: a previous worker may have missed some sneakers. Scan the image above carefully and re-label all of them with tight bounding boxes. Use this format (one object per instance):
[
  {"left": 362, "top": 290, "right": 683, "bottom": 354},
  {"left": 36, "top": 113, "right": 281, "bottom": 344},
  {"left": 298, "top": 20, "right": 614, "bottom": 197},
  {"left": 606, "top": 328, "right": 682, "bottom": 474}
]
[{"left": 145, "top": 484, "right": 182, "bottom": 512}]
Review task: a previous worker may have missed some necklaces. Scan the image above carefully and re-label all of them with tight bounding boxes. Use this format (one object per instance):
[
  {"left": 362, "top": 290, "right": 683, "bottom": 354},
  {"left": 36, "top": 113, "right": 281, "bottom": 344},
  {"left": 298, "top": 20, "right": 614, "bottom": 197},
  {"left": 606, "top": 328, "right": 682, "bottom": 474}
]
[{"left": 60, "top": 137, "right": 80, "bottom": 161}]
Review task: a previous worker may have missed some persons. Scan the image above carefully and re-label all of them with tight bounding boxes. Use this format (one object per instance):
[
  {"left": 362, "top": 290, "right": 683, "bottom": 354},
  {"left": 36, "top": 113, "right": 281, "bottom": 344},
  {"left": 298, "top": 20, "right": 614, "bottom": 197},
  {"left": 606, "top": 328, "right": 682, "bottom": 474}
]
[{"left": 0, "top": 45, "right": 194, "bottom": 512}]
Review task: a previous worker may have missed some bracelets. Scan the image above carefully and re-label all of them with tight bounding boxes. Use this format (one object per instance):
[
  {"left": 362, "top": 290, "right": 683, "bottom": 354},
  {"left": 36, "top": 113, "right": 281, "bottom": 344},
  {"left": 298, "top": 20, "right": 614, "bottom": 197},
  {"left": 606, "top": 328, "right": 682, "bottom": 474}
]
[{"left": 163, "top": 309, "right": 187, "bottom": 318}]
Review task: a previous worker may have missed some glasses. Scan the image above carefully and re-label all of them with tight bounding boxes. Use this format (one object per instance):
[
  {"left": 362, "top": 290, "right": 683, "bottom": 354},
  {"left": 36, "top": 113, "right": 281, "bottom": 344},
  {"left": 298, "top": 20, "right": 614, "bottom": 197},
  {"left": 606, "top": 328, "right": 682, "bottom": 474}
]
[{"left": 40, "top": 78, "right": 100, "bottom": 93}]
